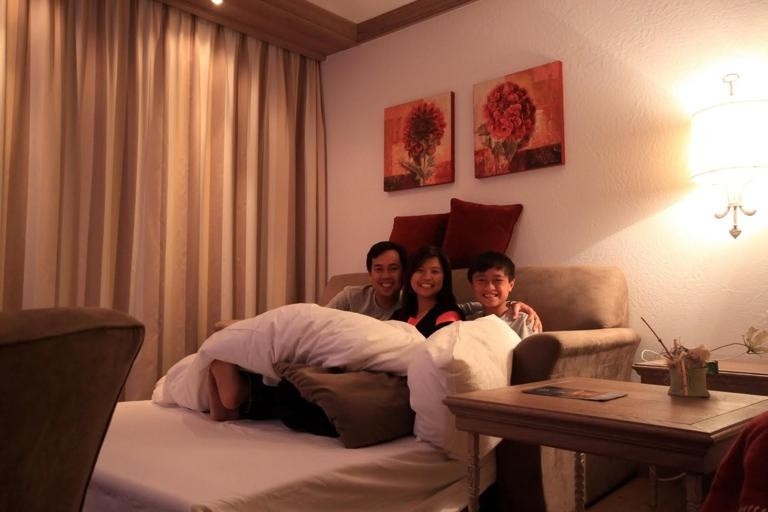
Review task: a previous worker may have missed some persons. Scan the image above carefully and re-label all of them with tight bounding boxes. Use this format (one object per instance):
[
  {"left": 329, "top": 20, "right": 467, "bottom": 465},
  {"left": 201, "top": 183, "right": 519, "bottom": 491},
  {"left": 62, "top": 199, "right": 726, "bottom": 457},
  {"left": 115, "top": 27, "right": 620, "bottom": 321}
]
[
  {"left": 208, "top": 248, "right": 466, "bottom": 422},
  {"left": 213, "top": 240, "right": 541, "bottom": 333},
  {"left": 275, "top": 249, "right": 541, "bottom": 439}
]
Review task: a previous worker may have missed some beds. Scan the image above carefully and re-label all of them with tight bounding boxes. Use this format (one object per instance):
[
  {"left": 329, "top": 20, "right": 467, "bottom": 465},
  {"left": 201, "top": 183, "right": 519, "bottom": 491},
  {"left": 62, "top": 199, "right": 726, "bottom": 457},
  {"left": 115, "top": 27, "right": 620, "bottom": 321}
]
[{"left": 88, "top": 260, "right": 643, "bottom": 510}]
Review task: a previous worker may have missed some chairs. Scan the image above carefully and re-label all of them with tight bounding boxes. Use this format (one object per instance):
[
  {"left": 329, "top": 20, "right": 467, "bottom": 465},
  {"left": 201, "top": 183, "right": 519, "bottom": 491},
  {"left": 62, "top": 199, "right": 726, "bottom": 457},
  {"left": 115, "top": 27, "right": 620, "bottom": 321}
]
[{"left": 0, "top": 300, "right": 147, "bottom": 511}]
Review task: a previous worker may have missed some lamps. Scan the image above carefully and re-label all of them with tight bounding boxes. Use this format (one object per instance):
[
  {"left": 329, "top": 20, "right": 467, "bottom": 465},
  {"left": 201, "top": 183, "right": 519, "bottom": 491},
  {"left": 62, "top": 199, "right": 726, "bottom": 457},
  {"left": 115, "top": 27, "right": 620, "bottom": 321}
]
[{"left": 705, "top": 66, "right": 761, "bottom": 242}]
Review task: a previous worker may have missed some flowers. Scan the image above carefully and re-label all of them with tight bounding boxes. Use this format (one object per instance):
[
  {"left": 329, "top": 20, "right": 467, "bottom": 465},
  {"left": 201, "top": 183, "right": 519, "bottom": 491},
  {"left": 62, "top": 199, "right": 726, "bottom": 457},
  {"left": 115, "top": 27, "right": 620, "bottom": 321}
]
[
  {"left": 640, "top": 315, "right": 768, "bottom": 371},
  {"left": 474, "top": 80, "right": 540, "bottom": 178},
  {"left": 396, "top": 100, "right": 450, "bottom": 188}
]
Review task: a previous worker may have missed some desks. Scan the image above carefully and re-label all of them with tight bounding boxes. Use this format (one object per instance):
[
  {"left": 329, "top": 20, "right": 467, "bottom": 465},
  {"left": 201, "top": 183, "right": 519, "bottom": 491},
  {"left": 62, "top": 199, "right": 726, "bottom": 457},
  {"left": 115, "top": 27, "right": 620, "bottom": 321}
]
[
  {"left": 626, "top": 352, "right": 767, "bottom": 511},
  {"left": 441, "top": 371, "right": 768, "bottom": 512}
]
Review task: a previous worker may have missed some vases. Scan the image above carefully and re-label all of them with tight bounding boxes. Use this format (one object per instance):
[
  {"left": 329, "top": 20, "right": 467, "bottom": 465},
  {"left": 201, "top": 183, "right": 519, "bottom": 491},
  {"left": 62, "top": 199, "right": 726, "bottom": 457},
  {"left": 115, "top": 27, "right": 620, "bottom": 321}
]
[{"left": 665, "top": 361, "right": 712, "bottom": 401}]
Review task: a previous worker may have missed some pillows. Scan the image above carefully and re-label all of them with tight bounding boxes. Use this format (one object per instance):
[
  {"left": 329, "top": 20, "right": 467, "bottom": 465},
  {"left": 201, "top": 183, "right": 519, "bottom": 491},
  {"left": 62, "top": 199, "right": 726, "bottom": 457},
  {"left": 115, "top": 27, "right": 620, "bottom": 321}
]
[
  {"left": 386, "top": 208, "right": 450, "bottom": 265},
  {"left": 439, "top": 196, "right": 524, "bottom": 270}
]
